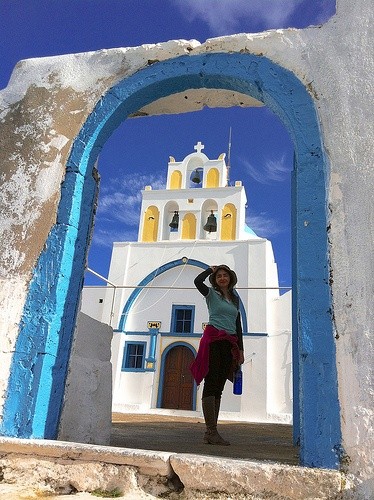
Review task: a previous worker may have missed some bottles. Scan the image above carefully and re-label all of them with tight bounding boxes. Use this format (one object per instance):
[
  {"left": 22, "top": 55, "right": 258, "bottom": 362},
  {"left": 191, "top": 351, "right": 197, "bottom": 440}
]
[{"left": 233, "top": 365, "right": 242, "bottom": 395}]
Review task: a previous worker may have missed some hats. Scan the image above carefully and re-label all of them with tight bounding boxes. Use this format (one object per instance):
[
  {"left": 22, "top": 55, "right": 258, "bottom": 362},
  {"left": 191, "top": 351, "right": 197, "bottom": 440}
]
[{"left": 209, "top": 265, "right": 237, "bottom": 288}]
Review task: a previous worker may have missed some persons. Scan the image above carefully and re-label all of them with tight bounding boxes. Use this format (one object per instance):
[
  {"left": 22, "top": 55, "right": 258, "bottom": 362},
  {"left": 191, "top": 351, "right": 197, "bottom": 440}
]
[{"left": 194, "top": 265, "right": 244, "bottom": 446}]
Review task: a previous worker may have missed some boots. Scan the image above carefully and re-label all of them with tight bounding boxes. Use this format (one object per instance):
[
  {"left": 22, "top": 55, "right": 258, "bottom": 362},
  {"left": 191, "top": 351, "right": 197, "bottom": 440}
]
[{"left": 202, "top": 395, "right": 231, "bottom": 445}]
